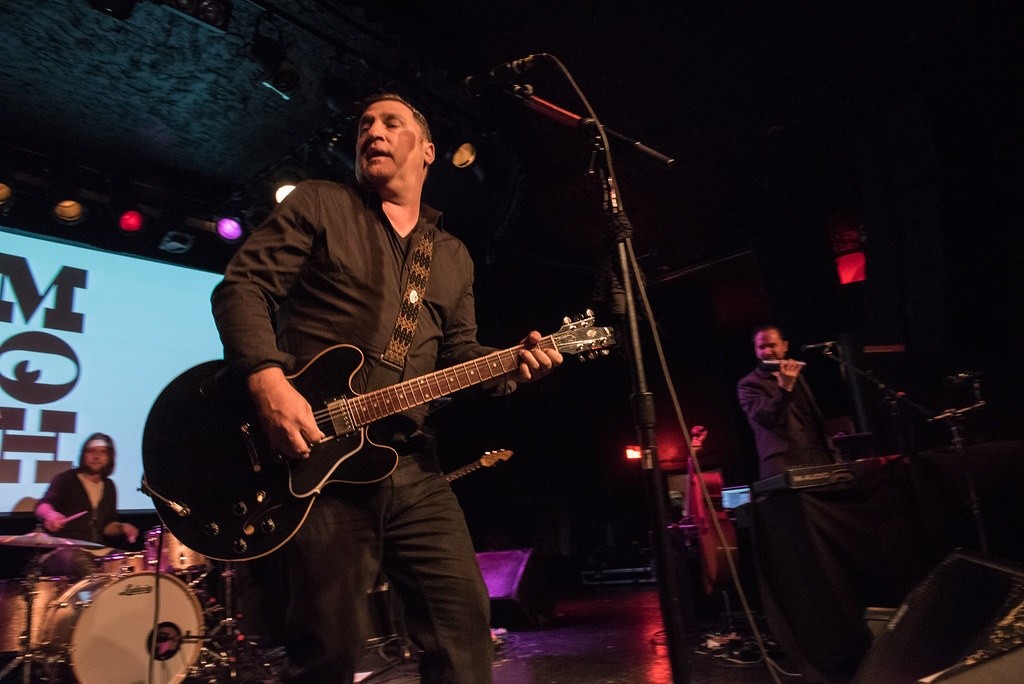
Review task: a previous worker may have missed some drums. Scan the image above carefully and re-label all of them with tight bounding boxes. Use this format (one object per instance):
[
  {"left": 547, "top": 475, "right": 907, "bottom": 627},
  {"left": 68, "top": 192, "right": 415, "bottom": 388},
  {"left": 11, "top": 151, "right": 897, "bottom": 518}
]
[
  {"left": 142, "top": 524, "right": 212, "bottom": 575},
  {"left": 40, "top": 571, "right": 207, "bottom": 684},
  {"left": 95, "top": 550, "right": 145, "bottom": 575},
  {"left": 0, "top": 573, "right": 71, "bottom": 658}
]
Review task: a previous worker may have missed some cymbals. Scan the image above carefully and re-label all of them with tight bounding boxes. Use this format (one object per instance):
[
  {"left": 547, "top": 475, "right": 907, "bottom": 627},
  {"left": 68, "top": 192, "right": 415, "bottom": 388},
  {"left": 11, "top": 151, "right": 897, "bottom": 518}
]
[{"left": 0, "top": 531, "right": 107, "bottom": 550}]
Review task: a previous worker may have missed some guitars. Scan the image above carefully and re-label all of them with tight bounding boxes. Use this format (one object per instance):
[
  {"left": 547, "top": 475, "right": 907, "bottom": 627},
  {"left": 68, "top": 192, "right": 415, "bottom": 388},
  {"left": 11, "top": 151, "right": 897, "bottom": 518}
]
[{"left": 139, "top": 309, "right": 620, "bottom": 566}]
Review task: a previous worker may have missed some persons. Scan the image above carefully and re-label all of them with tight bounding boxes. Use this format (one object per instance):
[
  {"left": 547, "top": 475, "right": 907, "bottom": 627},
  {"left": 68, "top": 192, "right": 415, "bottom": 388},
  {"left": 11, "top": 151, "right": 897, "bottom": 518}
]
[
  {"left": 209, "top": 94, "right": 564, "bottom": 684},
  {"left": 736, "top": 321, "right": 842, "bottom": 481},
  {"left": 33, "top": 433, "right": 140, "bottom": 549}
]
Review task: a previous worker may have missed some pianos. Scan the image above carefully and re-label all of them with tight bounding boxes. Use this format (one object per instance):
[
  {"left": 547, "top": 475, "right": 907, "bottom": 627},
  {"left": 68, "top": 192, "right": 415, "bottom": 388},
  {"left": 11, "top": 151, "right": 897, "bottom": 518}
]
[{"left": 750, "top": 435, "right": 1023, "bottom": 501}]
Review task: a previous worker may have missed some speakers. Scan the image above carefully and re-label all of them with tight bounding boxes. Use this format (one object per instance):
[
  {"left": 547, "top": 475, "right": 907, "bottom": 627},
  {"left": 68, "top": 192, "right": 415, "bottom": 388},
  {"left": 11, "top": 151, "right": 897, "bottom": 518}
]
[{"left": 841, "top": 554, "right": 1024, "bottom": 680}]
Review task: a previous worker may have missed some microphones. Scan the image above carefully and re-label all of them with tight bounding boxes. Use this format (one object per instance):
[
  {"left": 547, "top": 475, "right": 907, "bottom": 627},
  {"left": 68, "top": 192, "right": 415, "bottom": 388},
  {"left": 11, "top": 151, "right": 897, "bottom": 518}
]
[
  {"left": 800, "top": 339, "right": 839, "bottom": 356},
  {"left": 465, "top": 50, "right": 551, "bottom": 92}
]
